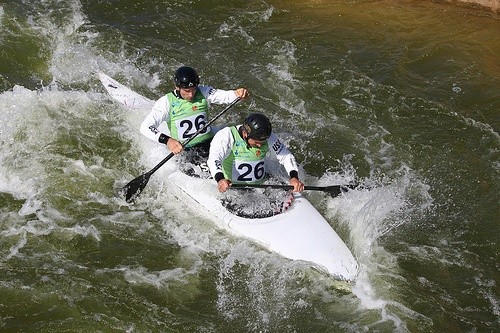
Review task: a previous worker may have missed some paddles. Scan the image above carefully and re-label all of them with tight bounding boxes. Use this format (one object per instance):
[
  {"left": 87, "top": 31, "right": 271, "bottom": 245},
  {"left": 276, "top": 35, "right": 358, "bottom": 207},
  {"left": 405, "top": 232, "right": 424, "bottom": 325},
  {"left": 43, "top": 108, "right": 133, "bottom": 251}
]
[
  {"left": 227, "top": 182, "right": 369, "bottom": 198},
  {"left": 120, "top": 95, "right": 243, "bottom": 204}
]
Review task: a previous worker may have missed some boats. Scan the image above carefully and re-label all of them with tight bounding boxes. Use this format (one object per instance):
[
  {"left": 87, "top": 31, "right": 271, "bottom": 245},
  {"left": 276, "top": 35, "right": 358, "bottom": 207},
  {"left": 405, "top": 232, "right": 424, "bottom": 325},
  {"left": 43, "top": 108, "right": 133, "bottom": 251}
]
[{"left": 98, "top": 72, "right": 360, "bottom": 283}]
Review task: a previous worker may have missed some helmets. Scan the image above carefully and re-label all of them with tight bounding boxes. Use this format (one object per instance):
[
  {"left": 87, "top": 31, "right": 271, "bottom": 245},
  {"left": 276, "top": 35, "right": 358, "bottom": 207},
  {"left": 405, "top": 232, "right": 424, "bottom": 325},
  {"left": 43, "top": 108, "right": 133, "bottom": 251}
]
[
  {"left": 174, "top": 67, "right": 200, "bottom": 88},
  {"left": 243, "top": 113, "right": 271, "bottom": 140}
]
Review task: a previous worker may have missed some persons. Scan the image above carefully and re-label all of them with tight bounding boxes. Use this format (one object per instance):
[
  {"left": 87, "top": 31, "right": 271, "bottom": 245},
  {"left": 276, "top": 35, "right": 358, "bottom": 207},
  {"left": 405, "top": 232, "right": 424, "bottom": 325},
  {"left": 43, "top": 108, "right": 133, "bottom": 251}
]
[
  {"left": 206, "top": 114, "right": 306, "bottom": 193},
  {"left": 139, "top": 66, "right": 250, "bottom": 171}
]
